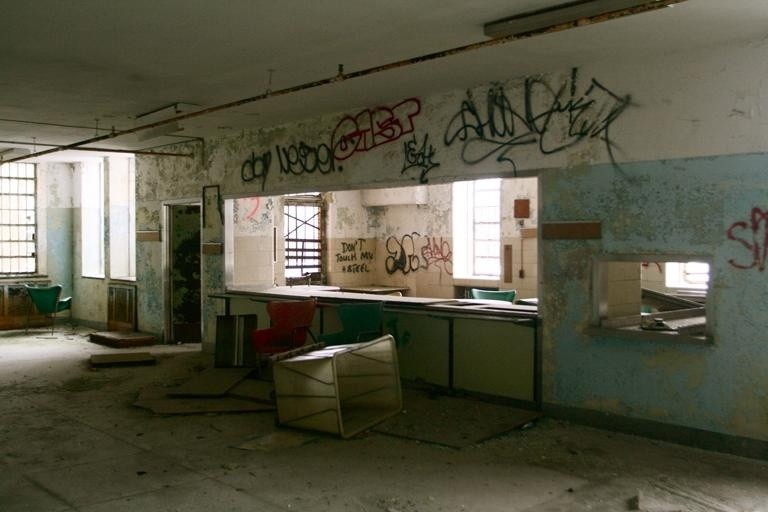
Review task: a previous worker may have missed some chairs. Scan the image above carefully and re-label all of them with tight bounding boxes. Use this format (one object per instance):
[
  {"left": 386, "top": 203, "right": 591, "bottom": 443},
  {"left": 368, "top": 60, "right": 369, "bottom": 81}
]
[
  {"left": 246, "top": 299, "right": 387, "bottom": 376},
  {"left": 19, "top": 281, "right": 75, "bottom": 338}
]
